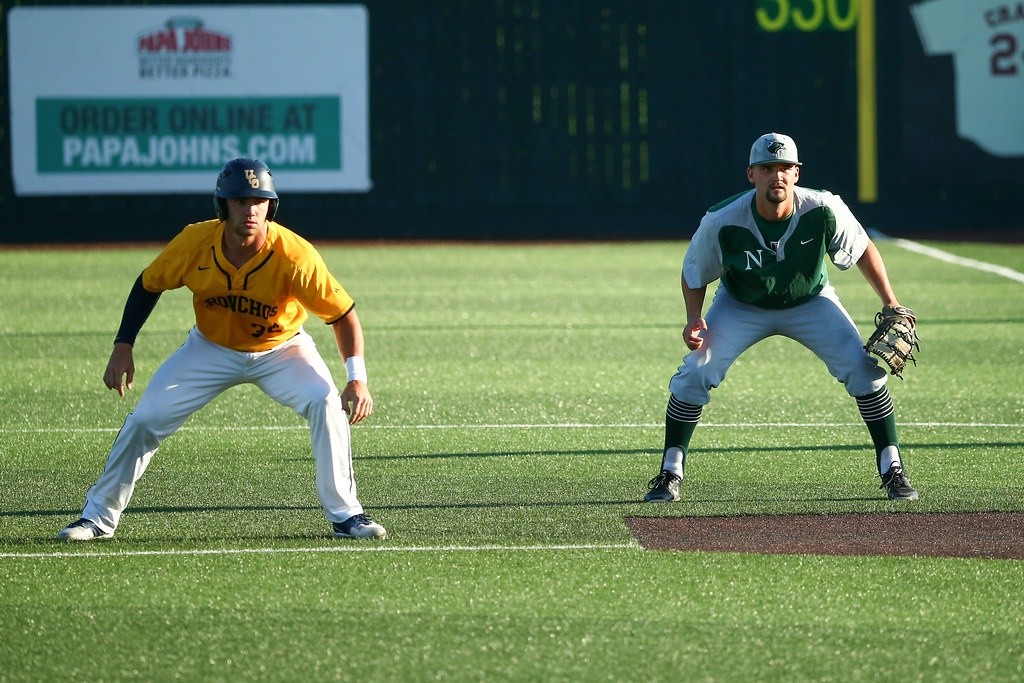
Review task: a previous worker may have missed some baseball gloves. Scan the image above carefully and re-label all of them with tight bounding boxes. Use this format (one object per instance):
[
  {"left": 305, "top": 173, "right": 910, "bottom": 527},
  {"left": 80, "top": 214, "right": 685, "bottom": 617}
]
[{"left": 865, "top": 306, "right": 917, "bottom": 375}]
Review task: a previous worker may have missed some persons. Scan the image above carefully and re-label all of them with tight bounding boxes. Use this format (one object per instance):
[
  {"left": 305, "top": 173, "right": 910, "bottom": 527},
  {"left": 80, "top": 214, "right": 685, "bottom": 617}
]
[
  {"left": 60, "top": 158, "right": 387, "bottom": 541},
  {"left": 645, "top": 132, "right": 919, "bottom": 500}
]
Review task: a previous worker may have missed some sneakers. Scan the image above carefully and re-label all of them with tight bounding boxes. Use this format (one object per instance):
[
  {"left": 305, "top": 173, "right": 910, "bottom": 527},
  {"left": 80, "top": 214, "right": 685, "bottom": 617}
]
[
  {"left": 876, "top": 460, "right": 917, "bottom": 502},
  {"left": 644, "top": 469, "right": 681, "bottom": 503},
  {"left": 59, "top": 515, "right": 115, "bottom": 541},
  {"left": 332, "top": 513, "right": 387, "bottom": 541}
]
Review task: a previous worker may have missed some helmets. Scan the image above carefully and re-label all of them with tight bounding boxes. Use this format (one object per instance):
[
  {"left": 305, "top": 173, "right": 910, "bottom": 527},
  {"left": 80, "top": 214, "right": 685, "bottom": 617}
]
[{"left": 213, "top": 159, "right": 279, "bottom": 223}]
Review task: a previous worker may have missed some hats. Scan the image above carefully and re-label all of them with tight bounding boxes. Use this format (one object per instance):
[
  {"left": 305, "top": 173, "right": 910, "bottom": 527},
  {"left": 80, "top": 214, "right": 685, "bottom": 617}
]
[{"left": 749, "top": 132, "right": 802, "bottom": 166}]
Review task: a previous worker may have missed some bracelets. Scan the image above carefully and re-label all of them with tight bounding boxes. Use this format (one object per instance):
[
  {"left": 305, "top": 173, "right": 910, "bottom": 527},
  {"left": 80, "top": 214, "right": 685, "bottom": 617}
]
[{"left": 345, "top": 356, "right": 368, "bottom": 382}]
